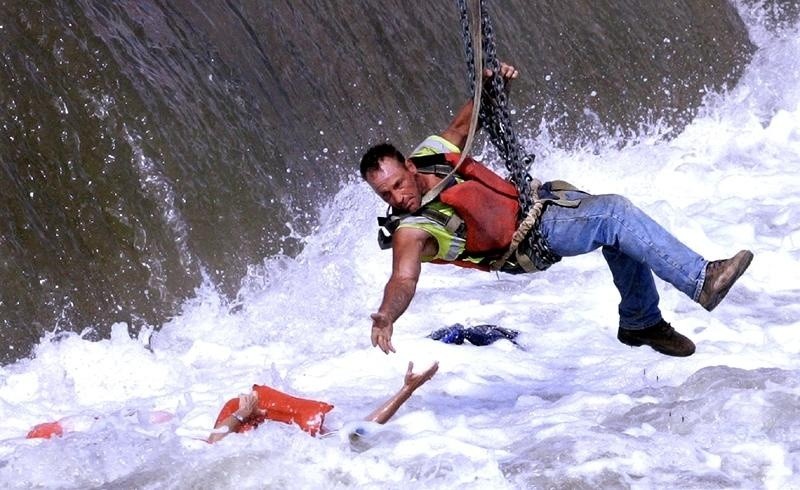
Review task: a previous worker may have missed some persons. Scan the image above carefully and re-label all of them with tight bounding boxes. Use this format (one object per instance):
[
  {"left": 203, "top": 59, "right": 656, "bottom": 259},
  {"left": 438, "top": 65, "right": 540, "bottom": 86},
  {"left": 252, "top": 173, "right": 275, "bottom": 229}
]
[
  {"left": 359, "top": 61, "right": 754, "bottom": 357},
  {"left": 202, "top": 360, "right": 445, "bottom": 446}
]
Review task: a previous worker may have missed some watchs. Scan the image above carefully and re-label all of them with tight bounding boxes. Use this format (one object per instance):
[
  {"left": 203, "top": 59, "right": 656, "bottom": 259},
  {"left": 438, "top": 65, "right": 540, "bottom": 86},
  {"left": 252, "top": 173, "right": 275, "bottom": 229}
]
[{"left": 231, "top": 412, "right": 245, "bottom": 423}]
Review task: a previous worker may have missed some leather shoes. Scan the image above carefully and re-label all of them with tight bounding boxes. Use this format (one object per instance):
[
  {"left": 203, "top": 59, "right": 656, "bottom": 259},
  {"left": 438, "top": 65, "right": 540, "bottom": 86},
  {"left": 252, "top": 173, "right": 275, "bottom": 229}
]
[
  {"left": 617, "top": 318, "right": 696, "bottom": 357},
  {"left": 697, "top": 249, "right": 754, "bottom": 312}
]
